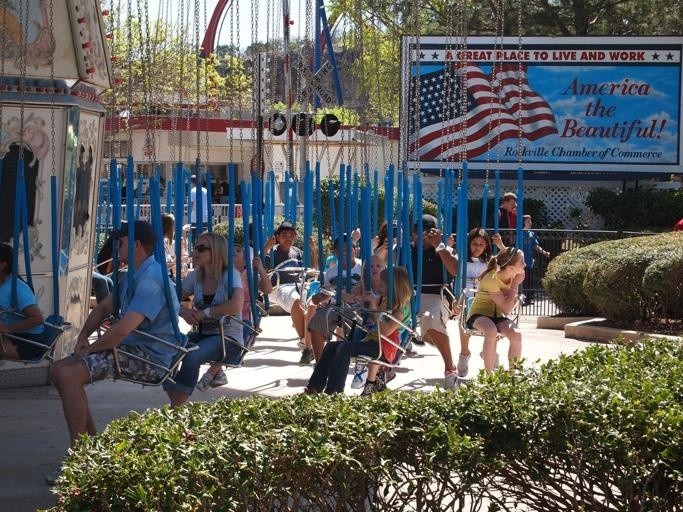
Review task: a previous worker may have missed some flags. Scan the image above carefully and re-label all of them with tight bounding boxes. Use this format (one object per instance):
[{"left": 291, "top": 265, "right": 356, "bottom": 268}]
[{"left": 407, "top": 62, "right": 559, "bottom": 162}]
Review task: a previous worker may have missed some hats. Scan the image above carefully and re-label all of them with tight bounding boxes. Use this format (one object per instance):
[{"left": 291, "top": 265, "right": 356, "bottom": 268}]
[{"left": 109, "top": 221, "right": 155, "bottom": 243}]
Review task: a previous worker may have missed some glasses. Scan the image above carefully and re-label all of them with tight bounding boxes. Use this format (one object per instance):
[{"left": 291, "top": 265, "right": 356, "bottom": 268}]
[{"left": 194, "top": 244, "right": 211, "bottom": 252}]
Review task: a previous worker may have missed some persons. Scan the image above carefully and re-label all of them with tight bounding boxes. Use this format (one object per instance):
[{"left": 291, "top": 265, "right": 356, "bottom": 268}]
[
  {"left": 1, "top": 176, "right": 549, "bottom": 484},
  {"left": 673, "top": 219, "right": 683, "bottom": 232}
]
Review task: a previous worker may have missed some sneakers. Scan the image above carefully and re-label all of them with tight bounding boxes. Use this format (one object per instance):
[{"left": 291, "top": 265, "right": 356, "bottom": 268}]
[
  {"left": 444, "top": 366, "right": 458, "bottom": 392},
  {"left": 458, "top": 351, "right": 471, "bottom": 377},
  {"left": 211, "top": 371, "right": 228, "bottom": 387},
  {"left": 196, "top": 373, "right": 212, "bottom": 392},
  {"left": 300, "top": 349, "right": 314, "bottom": 365},
  {"left": 351, "top": 365, "right": 396, "bottom": 395}
]
